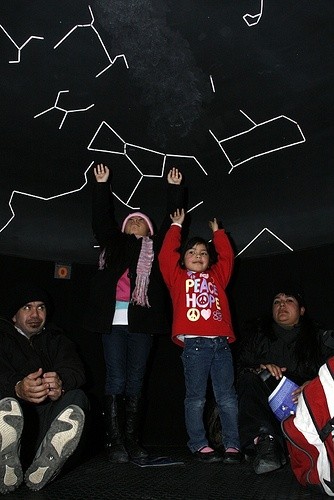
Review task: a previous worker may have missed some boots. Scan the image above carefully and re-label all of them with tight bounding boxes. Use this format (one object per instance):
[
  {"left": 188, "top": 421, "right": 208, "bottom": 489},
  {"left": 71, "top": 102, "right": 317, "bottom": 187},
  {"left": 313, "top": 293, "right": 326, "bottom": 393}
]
[{"left": 99, "top": 392, "right": 152, "bottom": 465}]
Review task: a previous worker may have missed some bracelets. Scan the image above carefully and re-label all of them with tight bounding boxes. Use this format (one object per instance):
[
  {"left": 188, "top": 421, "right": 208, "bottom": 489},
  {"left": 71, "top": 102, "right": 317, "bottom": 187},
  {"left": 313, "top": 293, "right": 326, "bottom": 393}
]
[{"left": 171, "top": 223, "right": 182, "bottom": 228}]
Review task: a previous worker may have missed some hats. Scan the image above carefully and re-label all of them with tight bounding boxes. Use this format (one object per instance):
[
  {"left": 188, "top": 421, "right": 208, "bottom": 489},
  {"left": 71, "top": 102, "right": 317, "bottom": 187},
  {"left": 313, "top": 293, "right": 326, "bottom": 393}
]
[
  {"left": 121, "top": 211, "right": 156, "bottom": 235},
  {"left": 8, "top": 281, "right": 50, "bottom": 319}
]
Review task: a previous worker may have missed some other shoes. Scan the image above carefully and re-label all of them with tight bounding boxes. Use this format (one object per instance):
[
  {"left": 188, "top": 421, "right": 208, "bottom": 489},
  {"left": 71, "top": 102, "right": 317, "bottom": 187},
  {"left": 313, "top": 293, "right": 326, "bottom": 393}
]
[
  {"left": 0, "top": 396, "right": 86, "bottom": 493},
  {"left": 236, "top": 431, "right": 288, "bottom": 474},
  {"left": 193, "top": 446, "right": 243, "bottom": 464}
]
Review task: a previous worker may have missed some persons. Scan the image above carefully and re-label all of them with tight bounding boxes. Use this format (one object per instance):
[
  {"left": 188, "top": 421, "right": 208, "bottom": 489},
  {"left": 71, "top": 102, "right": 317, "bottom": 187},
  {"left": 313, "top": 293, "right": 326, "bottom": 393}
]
[
  {"left": 158, "top": 208, "right": 243, "bottom": 463},
  {"left": 94, "top": 164, "right": 182, "bottom": 465},
  {"left": 232, "top": 288, "right": 334, "bottom": 474},
  {"left": 0, "top": 281, "right": 91, "bottom": 494}
]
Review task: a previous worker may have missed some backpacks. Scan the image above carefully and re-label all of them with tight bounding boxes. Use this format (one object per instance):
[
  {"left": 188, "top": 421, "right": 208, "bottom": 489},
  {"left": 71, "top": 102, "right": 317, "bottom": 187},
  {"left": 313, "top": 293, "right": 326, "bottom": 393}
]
[{"left": 281, "top": 355, "right": 334, "bottom": 498}]
